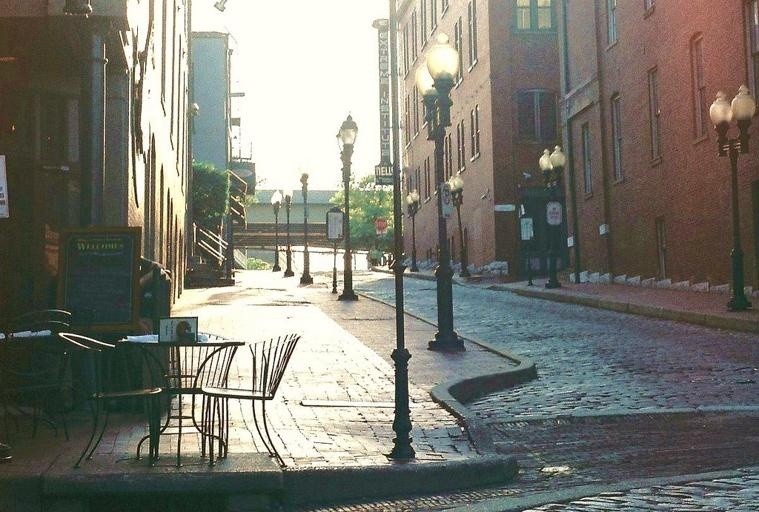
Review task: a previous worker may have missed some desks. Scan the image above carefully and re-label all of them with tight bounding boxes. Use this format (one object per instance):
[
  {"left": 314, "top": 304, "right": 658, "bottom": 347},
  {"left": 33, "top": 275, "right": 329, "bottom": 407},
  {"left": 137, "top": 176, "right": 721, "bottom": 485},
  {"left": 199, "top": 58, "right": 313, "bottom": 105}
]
[{"left": 116, "top": 331, "right": 246, "bottom": 467}]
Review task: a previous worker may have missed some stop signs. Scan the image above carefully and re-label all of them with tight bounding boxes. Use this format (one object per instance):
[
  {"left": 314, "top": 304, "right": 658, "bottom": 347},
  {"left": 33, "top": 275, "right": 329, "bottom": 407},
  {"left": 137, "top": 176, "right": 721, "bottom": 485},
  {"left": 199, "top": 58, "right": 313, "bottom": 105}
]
[{"left": 374, "top": 216, "right": 388, "bottom": 230}]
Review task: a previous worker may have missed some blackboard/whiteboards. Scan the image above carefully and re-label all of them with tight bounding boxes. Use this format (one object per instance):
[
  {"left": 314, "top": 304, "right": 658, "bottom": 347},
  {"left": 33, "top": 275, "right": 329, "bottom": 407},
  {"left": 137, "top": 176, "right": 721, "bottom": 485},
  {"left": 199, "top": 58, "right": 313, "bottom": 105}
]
[{"left": 55, "top": 227, "right": 141, "bottom": 331}]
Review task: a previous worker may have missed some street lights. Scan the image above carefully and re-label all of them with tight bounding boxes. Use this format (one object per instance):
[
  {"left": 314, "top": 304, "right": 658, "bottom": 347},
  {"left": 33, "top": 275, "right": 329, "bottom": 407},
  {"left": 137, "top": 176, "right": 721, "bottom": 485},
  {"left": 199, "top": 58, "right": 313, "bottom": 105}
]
[
  {"left": 296, "top": 159, "right": 316, "bottom": 284},
  {"left": 443, "top": 174, "right": 471, "bottom": 277},
  {"left": 337, "top": 115, "right": 361, "bottom": 302},
  {"left": 406, "top": 188, "right": 421, "bottom": 273},
  {"left": 283, "top": 184, "right": 294, "bottom": 277},
  {"left": 708, "top": 85, "right": 757, "bottom": 310},
  {"left": 271, "top": 189, "right": 283, "bottom": 272},
  {"left": 413, "top": 32, "right": 467, "bottom": 353},
  {"left": 539, "top": 145, "right": 568, "bottom": 291}
]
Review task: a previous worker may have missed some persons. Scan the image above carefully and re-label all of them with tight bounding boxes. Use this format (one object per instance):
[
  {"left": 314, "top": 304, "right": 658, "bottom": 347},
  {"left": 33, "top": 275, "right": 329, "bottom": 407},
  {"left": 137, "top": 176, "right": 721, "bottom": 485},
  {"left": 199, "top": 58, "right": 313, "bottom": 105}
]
[{"left": 366, "top": 245, "right": 392, "bottom": 270}]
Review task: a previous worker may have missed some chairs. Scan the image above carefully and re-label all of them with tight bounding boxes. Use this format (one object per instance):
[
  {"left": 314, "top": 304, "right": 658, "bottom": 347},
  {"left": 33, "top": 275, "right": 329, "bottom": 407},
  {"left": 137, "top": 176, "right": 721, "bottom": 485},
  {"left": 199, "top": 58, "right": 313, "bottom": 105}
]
[
  {"left": 198, "top": 332, "right": 303, "bottom": 470},
  {"left": 0, "top": 311, "right": 163, "bottom": 469}
]
